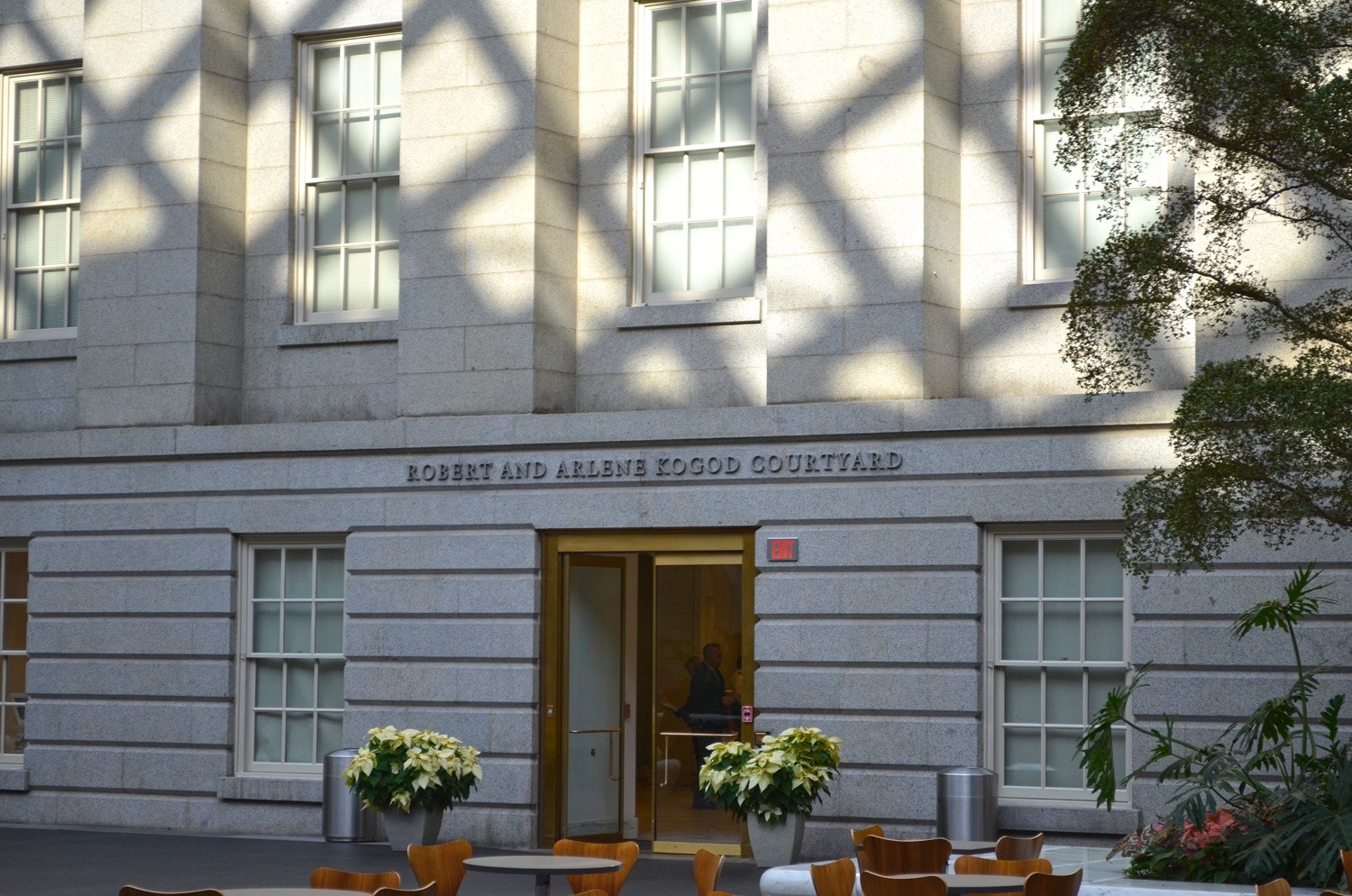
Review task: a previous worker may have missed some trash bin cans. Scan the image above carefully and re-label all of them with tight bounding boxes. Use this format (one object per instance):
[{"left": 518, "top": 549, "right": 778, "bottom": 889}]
[
  {"left": 323, "top": 748, "right": 379, "bottom": 842},
  {"left": 1004, "top": 764, "right": 1057, "bottom": 788},
  {"left": 936, "top": 766, "right": 999, "bottom": 843}
]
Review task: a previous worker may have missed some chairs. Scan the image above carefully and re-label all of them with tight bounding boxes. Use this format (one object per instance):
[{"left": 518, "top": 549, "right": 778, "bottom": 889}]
[
  {"left": 856, "top": 833, "right": 951, "bottom": 873},
  {"left": 1024, "top": 869, "right": 1083, "bottom": 896},
  {"left": 851, "top": 823, "right": 884, "bottom": 853},
  {"left": 407, "top": 841, "right": 471, "bottom": 896},
  {"left": 952, "top": 856, "right": 1053, "bottom": 879},
  {"left": 554, "top": 839, "right": 639, "bottom": 894},
  {"left": 372, "top": 882, "right": 437, "bottom": 896},
  {"left": 694, "top": 848, "right": 725, "bottom": 896},
  {"left": 858, "top": 871, "right": 946, "bottom": 896},
  {"left": 810, "top": 858, "right": 856, "bottom": 896},
  {"left": 995, "top": 833, "right": 1043, "bottom": 860},
  {"left": 119, "top": 884, "right": 222, "bottom": 896},
  {"left": 309, "top": 866, "right": 401, "bottom": 892}
]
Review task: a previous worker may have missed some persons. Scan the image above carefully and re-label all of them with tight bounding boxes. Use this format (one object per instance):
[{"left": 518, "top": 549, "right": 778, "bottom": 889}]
[
  {"left": 688, "top": 643, "right": 735, "bottom": 810},
  {"left": 732, "top": 656, "right": 743, "bottom": 724},
  {"left": 674, "top": 657, "right": 700, "bottom": 728}
]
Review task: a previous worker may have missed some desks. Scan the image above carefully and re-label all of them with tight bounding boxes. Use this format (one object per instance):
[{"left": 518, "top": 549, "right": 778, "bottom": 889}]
[
  {"left": 947, "top": 840, "right": 997, "bottom": 856},
  {"left": 886, "top": 874, "right": 1028, "bottom": 896},
  {"left": 458, "top": 856, "right": 623, "bottom": 896}
]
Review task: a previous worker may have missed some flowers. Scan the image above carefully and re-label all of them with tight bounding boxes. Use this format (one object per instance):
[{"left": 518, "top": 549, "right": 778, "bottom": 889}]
[
  {"left": 339, "top": 725, "right": 484, "bottom": 813},
  {"left": 697, "top": 720, "right": 838, "bottom": 826}
]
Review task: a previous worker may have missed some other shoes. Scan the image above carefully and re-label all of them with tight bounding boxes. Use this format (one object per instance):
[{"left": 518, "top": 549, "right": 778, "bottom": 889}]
[{"left": 692, "top": 796, "right": 716, "bottom": 810}]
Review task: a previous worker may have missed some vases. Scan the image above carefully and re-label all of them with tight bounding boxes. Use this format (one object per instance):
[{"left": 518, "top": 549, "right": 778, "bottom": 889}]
[
  {"left": 745, "top": 810, "right": 808, "bottom": 867},
  {"left": 375, "top": 804, "right": 445, "bottom": 851}
]
